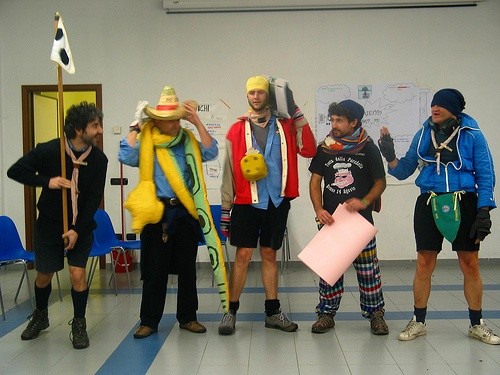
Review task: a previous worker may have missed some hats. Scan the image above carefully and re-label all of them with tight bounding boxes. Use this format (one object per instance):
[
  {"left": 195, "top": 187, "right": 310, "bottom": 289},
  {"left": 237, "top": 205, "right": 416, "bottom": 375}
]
[{"left": 142, "top": 86, "right": 198, "bottom": 120}]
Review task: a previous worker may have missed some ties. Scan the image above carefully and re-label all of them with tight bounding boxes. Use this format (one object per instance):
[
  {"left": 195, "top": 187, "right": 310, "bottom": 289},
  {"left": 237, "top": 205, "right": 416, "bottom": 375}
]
[{"left": 64, "top": 137, "right": 92, "bottom": 225}]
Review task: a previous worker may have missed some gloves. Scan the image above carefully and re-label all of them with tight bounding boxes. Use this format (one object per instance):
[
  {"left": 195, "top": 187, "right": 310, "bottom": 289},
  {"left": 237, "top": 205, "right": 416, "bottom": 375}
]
[
  {"left": 130, "top": 101, "right": 148, "bottom": 131},
  {"left": 292, "top": 104, "right": 304, "bottom": 121},
  {"left": 220, "top": 208, "right": 231, "bottom": 238}
]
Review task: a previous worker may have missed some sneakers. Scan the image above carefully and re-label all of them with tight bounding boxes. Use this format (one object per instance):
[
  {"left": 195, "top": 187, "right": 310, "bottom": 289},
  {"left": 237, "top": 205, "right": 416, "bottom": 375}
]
[
  {"left": 179, "top": 321, "right": 206, "bottom": 333},
  {"left": 369, "top": 309, "right": 389, "bottom": 334},
  {"left": 468, "top": 319, "right": 500, "bottom": 345},
  {"left": 134, "top": 325, "right": 158, "bottom": 339},
  {"left": 21, "top": 308, "right": 49, "bottom": 340},
  {"left": 265, "top": 309, "right": 298, "bottom": 332},
  {"left": 218, "top": 310, "right": 236, "bottom": 335},
  {"left": 399, "top": 314, "right": 427, "bottom": 341},
  {"left": 68, "top": 316, "right": 89, "bottom": 349},
  {"left": 312, "top": 314, "right": 335, "bottom": 333}
]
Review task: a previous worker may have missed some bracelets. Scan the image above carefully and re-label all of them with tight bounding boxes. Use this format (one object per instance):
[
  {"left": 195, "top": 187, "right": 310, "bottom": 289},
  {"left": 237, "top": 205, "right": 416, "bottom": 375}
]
[{"left": 363, "top": 198, "right": 369, "bottom": 206}]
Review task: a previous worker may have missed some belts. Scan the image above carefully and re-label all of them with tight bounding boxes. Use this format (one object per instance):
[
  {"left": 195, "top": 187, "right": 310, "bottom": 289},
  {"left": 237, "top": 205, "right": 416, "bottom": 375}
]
[{"left": 161, "top": 198, "right": 181, "bottom": 206}]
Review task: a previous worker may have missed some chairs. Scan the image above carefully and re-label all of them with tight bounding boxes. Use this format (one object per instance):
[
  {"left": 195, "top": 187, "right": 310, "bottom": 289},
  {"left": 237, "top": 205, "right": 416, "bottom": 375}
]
[
  {"left": 0, "top": 215, "right": 63, "bottom": 319},
  {"left": 64, "top": 209, "right": 140, "bottom": 296},
  {"left": 197, "top": 204, "right": 231, "bottom": 286}
]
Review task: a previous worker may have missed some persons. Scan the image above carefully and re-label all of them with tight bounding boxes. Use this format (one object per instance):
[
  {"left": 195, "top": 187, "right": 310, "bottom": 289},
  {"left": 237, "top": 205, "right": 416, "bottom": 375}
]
[
  {"left": 219, "top": 76, "right": 317, "bottom": 334},
  {"left": 306, "top": 99, "right": 388, "bottom": 335},
  {"left": 377, "top": 88, "right": 500, "bottom": 346},
  {"left": 117, "top": 87, "right": 218, "bottom": 339},
  {"left": 6, "top": 101, "right": 109, "bottom": 349}
]
